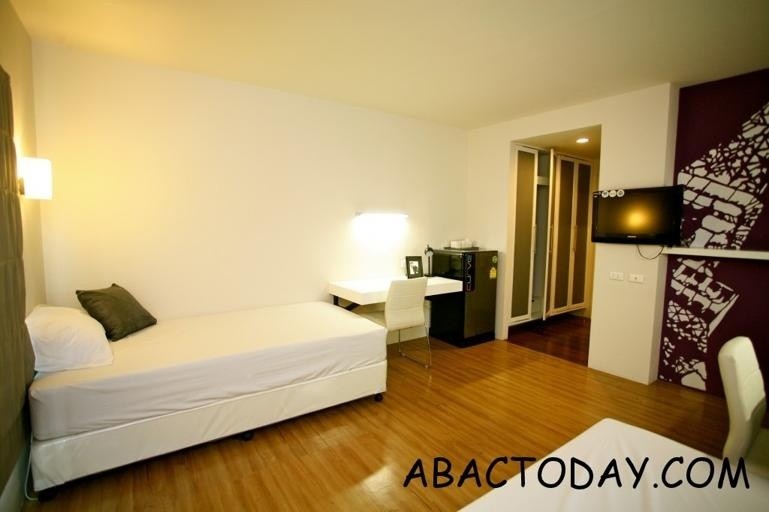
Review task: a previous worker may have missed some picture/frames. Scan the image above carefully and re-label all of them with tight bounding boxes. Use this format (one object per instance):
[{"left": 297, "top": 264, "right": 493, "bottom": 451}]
[{"left": 406, "top": 256, "right": 423, "bottom": 279}]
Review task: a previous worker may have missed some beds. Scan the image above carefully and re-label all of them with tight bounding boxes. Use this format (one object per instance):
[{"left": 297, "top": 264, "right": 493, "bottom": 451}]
[{"left": 26, "top": 302, "right": 388, "bottom": 500}]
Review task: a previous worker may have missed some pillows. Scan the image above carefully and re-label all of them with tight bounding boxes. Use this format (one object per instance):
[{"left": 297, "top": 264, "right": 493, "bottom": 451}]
[{"left": 24, "top": 283, "right": 157, "bottom": 373}]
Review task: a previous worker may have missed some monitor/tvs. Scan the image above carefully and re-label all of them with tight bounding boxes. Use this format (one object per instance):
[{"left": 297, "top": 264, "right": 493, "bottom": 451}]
[{"left": 591, "top": 183, "right": 684, "bottom": 246}]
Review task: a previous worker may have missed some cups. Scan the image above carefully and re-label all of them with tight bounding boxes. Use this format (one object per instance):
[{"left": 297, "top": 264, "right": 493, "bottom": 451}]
[{"left": 451, "top": 241, "right": 472, "bottom": 248}]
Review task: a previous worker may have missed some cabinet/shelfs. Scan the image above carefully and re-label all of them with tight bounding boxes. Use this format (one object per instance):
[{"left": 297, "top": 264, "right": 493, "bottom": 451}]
[{"left": 507, "top": 143, "right": 594, "bottom": 327}]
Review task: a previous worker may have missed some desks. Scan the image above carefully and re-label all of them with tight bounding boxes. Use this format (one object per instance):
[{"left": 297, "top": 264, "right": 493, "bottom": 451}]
[{"left": 331, "top": 274, "right": 463, "bottom": 309}]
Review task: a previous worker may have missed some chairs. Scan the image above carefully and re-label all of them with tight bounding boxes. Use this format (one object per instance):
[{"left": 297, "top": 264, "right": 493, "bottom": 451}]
[
  {"left": 355, "top": 277, "right": 431, "bottom": 368},
  {"left": 719, "top": 336, "right": 769, "bottom": 477}
]
